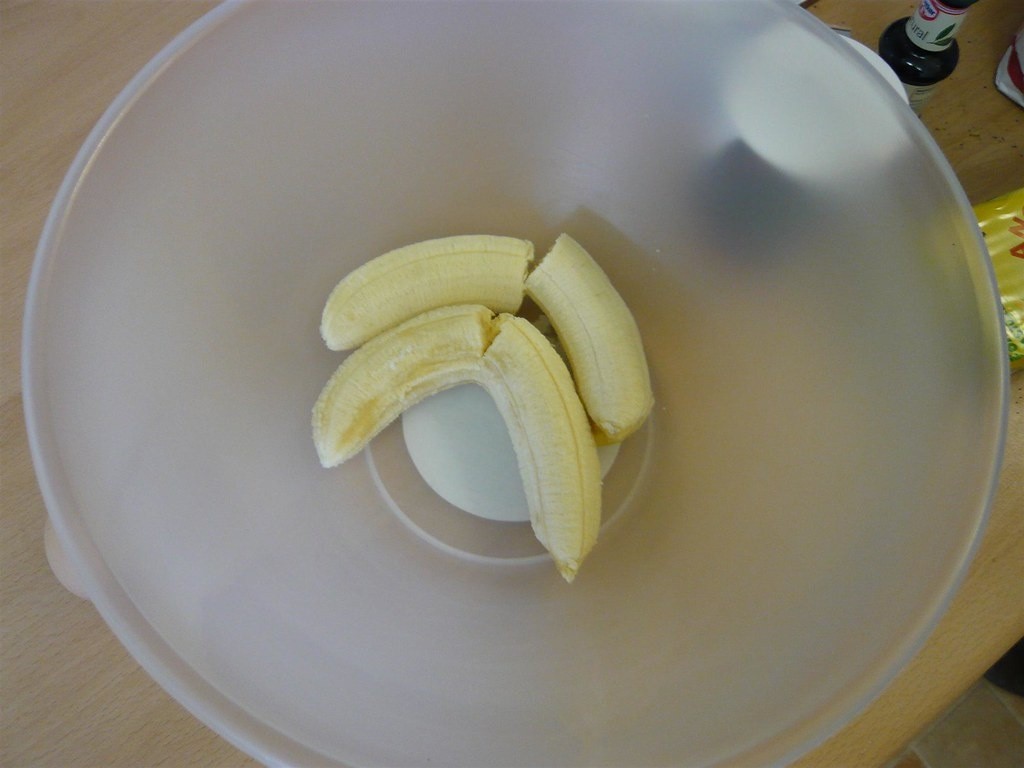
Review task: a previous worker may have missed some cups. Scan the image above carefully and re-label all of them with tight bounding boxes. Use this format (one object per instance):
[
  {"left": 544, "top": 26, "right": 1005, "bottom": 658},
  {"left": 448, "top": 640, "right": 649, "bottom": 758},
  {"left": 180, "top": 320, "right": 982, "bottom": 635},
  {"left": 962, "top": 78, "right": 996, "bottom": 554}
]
[{"left": 685, "top": 22, "right": 909, "bottom": 237}]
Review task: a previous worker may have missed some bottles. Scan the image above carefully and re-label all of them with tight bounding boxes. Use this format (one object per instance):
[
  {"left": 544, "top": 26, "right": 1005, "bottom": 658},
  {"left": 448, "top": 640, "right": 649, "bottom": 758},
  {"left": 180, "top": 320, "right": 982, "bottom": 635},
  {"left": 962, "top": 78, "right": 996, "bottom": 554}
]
[{"left": 875, "top": 0, "right": 978, "bottom": 116}]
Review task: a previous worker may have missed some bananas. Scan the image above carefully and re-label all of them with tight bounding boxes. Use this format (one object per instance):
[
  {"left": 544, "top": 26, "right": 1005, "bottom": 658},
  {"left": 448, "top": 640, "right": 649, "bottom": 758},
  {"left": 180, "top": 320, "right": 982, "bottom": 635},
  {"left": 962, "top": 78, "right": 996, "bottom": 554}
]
[
  {"left": 319, "top": 234, "right": 536, "bottom": 351},
  {"left": 310, "top": 304, "right": 601, "bottom": 581},
  {"left": 522, "top": 232, "right": 655, "bottom": 444}
]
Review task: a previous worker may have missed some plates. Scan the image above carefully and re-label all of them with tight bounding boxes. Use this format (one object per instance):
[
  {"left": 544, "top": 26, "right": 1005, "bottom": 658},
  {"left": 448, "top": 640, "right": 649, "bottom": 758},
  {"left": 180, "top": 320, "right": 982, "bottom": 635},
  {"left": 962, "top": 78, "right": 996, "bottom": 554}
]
[{"left": 19, "top": 0, "right": 1011, "bottom": 768}]
